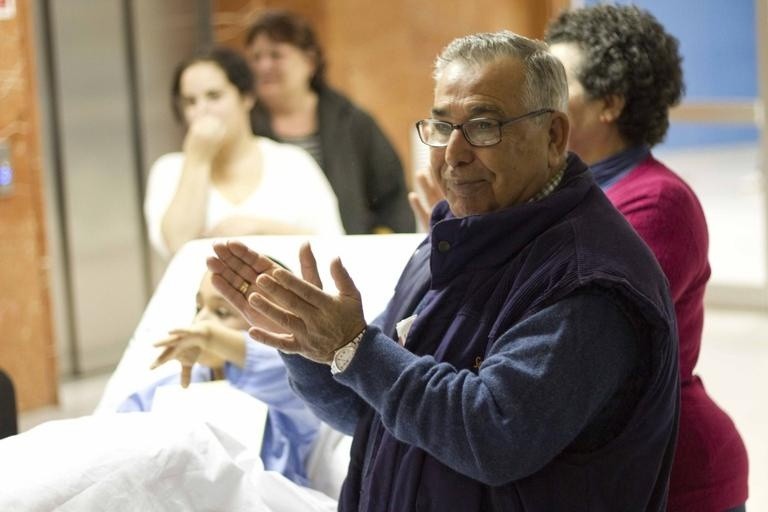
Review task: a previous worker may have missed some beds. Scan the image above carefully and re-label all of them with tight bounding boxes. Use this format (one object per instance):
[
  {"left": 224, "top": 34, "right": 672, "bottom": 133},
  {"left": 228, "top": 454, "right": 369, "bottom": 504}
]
[{"left": 91, "top": 234, "right": 468, "bottom": 512}]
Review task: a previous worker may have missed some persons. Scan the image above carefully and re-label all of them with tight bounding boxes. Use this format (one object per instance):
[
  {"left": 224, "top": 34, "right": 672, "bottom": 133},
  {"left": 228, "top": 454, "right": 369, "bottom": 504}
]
[
  {"left": 116, "top": 268, "right": 321, "bottom": 488},
  {"left": 240, "top": 11, "right": 418, "bottom": 235},
  {"left": 540, "top": 2, "right": 751, "bottom": 512},
  {"left": 142, "top": 39, "right": 346, "bottom": 263},
  {"left": 201, "top": 29, "right": 681, "bottom": 508}
]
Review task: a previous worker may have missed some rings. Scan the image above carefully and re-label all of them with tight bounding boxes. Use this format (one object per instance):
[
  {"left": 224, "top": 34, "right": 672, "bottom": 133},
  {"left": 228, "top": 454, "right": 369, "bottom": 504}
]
[{"left": 238, "top": 281, "right": 249, "bottom": 295}]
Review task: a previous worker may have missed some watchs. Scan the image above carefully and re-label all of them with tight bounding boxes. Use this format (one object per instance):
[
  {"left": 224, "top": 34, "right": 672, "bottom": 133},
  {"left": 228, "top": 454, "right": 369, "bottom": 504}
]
[{"left": 328, "top": 326, "right": 368, "bottom": 376}]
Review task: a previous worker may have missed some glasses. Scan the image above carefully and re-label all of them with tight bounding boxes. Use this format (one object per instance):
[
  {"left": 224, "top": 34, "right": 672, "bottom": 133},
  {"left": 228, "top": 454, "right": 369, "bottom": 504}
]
[{"left": 416, "top": 107, "right": 554, "bottom": 147}]
